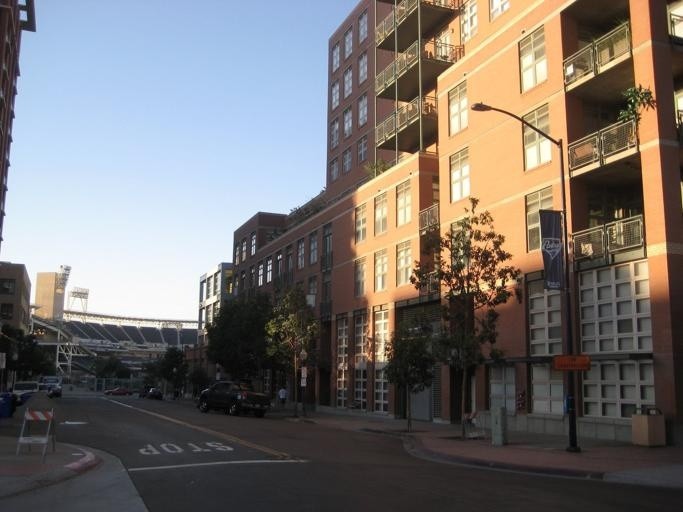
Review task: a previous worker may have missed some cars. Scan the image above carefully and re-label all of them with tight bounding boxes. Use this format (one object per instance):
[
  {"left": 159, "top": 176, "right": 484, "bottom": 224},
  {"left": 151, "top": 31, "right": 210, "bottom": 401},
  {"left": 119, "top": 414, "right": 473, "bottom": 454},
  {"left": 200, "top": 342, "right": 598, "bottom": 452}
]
[
  {"left": 104, "top": 387, "right": 133, "bottom": 396},
  {"left": 146, "top": 386, "right": 163, "bottom": 400},
  {"left": 12, "top": 373, "right": 63, "bottom": 405}
]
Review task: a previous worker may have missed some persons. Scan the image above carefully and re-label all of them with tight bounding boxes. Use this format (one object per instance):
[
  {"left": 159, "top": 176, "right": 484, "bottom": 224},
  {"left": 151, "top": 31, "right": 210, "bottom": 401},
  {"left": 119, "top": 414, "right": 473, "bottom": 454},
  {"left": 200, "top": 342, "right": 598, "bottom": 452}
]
[{"left": 277, "top": 386, "right": 286, "bottom": 408}]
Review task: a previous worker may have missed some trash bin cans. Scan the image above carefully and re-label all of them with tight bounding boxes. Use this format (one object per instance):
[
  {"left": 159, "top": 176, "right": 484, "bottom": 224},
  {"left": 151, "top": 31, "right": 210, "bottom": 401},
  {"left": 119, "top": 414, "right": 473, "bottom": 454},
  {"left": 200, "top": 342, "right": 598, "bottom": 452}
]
[
  {"left": 0, "top": 393, "right": 18, "bottom": 418},
  {"left": 631, "top": 407, "right": 666, "bottom": 447}
]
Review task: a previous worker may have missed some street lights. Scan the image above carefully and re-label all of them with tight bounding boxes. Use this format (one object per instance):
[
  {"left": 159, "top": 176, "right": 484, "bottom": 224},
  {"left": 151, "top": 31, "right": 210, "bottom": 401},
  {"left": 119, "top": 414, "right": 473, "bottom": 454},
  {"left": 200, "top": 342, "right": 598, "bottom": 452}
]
[{"left": 471, "top": 101, "right": 582, "bottom": 454}]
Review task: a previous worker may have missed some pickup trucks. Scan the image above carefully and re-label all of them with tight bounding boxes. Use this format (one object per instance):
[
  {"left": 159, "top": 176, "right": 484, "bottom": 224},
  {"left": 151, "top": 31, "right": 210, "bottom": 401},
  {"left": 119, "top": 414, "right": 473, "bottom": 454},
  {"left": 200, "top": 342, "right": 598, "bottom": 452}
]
[{"left": 197, "top": 380, "right": 271, "bottom": 419}]
